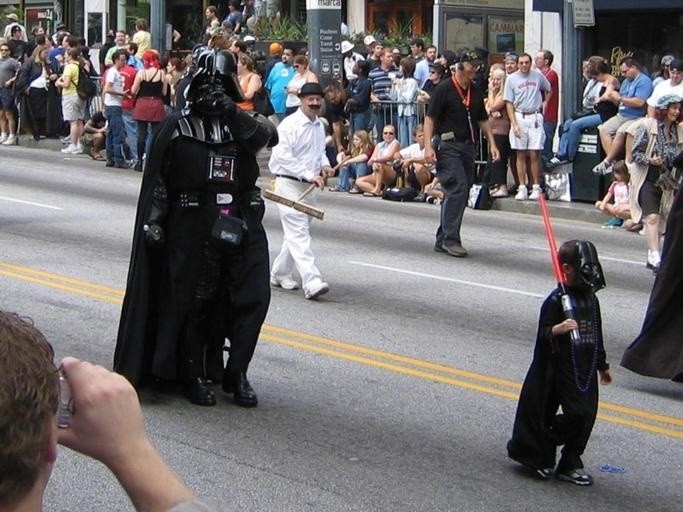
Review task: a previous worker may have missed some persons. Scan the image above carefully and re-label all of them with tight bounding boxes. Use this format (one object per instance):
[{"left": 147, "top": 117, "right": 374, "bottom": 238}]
[
  {"left": 422, "top": 50, "right": 501, "bottom": 257},
  {"left": 1, "top": 310, "right": 209, "bottom": 512},
  {"left": 619, "top": 152, "right": 683, "bottom": 383},
  {"left": 506, "top": 238, "right": 612, "bottom": 486},
  {"left": 1, "top": 1, "right": 683, "bottom": 236},
  {"left": 627, "top": 94, "right": 683, "bottom": 273},
  {"left": 268, "top": 83, "right": 336, "bottom": 300},
  {"left": 113, "top": 43, "right": 280, "bottom": 409}
]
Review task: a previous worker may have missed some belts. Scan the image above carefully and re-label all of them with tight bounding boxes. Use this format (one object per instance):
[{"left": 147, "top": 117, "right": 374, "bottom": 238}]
[
  {"left": 523, "top": 110, "right": 541, "bottom": 115},
  {"left": 276, "top": 174, "right": 312, "bottom": 183}
]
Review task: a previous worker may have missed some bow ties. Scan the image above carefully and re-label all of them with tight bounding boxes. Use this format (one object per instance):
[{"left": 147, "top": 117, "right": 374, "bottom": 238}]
[{"left": 515, "top": 188, "right": 527, "bottom": 200}]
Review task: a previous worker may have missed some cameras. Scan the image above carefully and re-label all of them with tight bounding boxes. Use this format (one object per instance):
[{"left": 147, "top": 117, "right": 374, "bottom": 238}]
[{"left": 56, "top": 368, "right": 73, "bottom": 428}]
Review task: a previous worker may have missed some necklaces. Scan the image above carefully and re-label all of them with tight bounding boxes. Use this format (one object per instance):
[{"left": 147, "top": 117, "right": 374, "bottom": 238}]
[{"left": 569, "top": 287, "right": 600, "bottom": 394}]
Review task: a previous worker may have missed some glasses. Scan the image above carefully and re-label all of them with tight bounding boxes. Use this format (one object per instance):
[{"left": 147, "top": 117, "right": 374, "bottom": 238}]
[
  {"left": 2, "top": 48, "right": 9, "bottom": 52},
  {"left": 429, "top": 70, "right": 436, "bottom": 74},
  {"left": 292, "top": 65, "right": 299, "bottom": 69},
  {"left": 383, "top": 132, "right": 393, "bottom": 135}
]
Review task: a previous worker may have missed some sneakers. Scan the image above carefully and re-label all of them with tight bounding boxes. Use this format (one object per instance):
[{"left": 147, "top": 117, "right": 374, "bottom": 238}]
[
  {"left": 442, "top": 241, "right": 467, "bottom": 257},
  {"left": 426, "top": 196, "right": 441, "bottom": 204},
  {"left": 610, "top": 217, "right": 624, "bottom": 228},
  {"left": 60, "top": 145, "right": 76, "bottom": 153},
  {"left": 646, "top": 261, "right": 653, "bottom": 269},
  {"left": 591, "top": 161, "right": 608, "bottom": 175},
  {"left": 348, "top": 188, "right": 363, "bottom": 194},
  {"left": 435, "top": 243, "right": 447, "bottom": 253},
  {"left": 491, "top": 190, "right": 508, "bottom": 198},
  {"left": 555, "top": 464, "right": 593, "bottom": 485},
  {"left": 546, "top": 156, "right": 568, "bottom": 167},
  {"left": 528, "top": 188, "right": 541, "bottom": 199},
  {"left": 271, "top": 273, "right": 299, "bottom": 290},
  {"left": 105, "top": 160, "right": 114, "bottom": 167},
  {"left": 304, "top": 281, "right": 328, "bottom": 300},
  {"left": 530, "top": 455, "right": 553, "bottom": 478},
  {"left": 70, "top": 146, "right": 85, "bottom": 154},
  {"left": 601, "top": 218, "right": 617, "bottom": 228},
  {"left": 115, "top": 160, "right": 130, "bottom": 169},
  {"left": 653, "top": 262, "right": 663, "bottom": 274},
  {"left": 329, "top": 186, "right": 348, "bottom": 192},
  {"left": 60, "top": 135, "right": 71, "bottom": 144},
  {"left": 414, "top": 192, "right": 425, "bottom": 201},
  {"left": 2, "top": 137, "right": 15, "bottom": 145},
  {"left": 603, "top": 162, "right": 615, "bottom": 175},
  {"left": 0, "top": 136, "right": 7, "bottom": 144}
]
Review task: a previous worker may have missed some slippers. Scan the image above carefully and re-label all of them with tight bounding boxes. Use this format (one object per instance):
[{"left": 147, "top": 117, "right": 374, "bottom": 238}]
[{"left": 363, "top": 191, "right": 382, "bottom": 197}]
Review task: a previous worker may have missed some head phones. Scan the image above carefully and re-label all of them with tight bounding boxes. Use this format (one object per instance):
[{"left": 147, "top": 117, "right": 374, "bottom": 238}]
[{"left": 458, "top": 50, "right": 468, "bottom": 71}]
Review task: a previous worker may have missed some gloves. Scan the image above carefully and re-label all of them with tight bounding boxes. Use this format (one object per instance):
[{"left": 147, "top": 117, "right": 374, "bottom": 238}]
[
  {"left": 144, "top": 188, "right": 166, "bottom": 245},
  {"left": 196, "top": 90, "right": 258, "bottom": 141}
]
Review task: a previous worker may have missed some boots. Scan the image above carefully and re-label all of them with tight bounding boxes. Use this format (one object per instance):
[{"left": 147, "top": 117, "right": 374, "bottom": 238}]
[
  {"left": 221, "top": 345, "right": 257, "bottom": 407},
  {"left": 184, "top": 341, "right": 217, "bottom": 406}
]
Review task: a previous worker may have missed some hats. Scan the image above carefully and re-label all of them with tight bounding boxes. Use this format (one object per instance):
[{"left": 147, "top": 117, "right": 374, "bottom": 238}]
[
  {"left": 453, "top": 50, "right": 483, "bottom": 66},
  {"left": 5, "top": 13, "right": 17, "bottom": 21},
  {"left": 363, "top": 35, "right": 375, "bottom": 45},
  {"left": 651, "top": 91, "right": 683, "bottom": 106},
  {"left": 296, "top": 83, "right": 325, "bottom": 98},
  {"left": 669, "top": 59, "right": 683, "bottom": 72},
  {"left": 506, "top": 52, "right": 518, "bottom": 63},
  {"left": 340, "top": 41, "right": 354, "bottom": 54},
  {"left": 428, "top": 63, "right": 446, "bottom": 74}
]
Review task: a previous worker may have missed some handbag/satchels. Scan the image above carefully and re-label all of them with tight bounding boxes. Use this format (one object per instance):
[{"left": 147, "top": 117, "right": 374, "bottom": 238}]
[{"left": 468, "top": 185, "right": 492, "bottom": 209}]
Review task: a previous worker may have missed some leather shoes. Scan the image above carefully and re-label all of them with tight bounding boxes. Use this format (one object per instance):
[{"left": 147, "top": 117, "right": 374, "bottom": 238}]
[
  {"left": 33, "top": 133, "right": 40, "bottom": 141},
  {"left": 46, "top": 134, "right": 58, "bottom": 139}
]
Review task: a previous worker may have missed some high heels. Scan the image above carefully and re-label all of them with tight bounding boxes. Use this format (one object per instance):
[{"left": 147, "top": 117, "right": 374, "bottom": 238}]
[{"left": 134, "top": 159, "right": 142, "bottom": 171}]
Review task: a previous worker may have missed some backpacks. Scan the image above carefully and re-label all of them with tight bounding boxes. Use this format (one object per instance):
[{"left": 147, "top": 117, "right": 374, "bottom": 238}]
[
  {"left": 382, "top": 186, "right": 418, "bottom": 202},
  {"left": 69, "top": 62, "right": 96, "bottom": 105}
]
